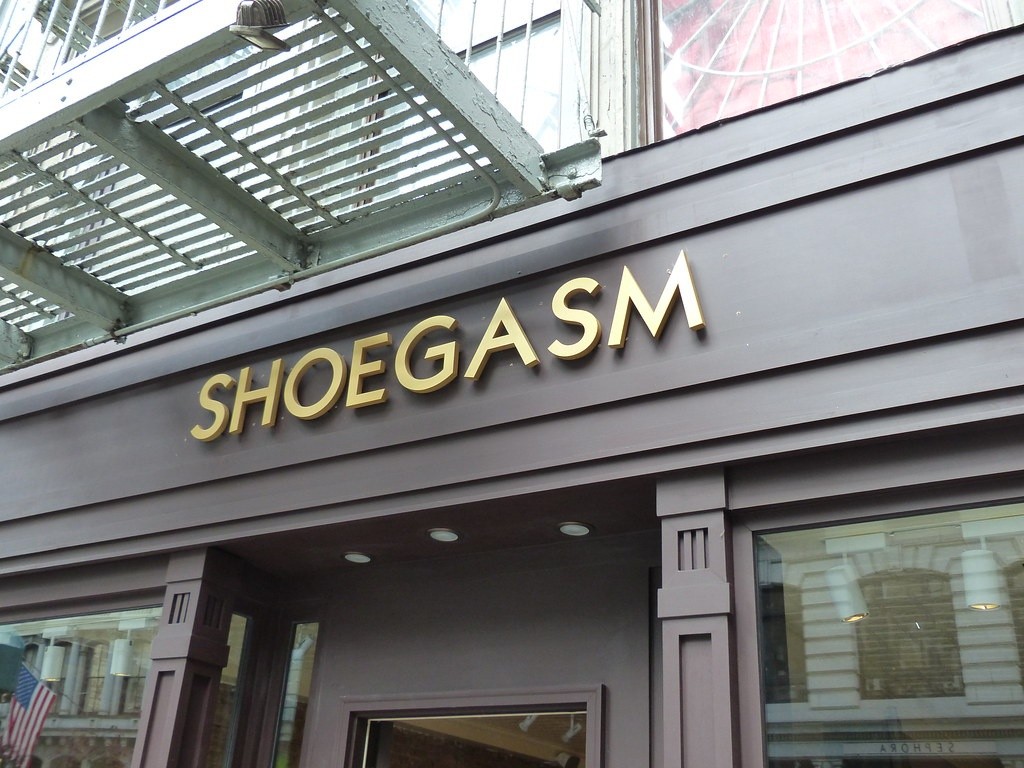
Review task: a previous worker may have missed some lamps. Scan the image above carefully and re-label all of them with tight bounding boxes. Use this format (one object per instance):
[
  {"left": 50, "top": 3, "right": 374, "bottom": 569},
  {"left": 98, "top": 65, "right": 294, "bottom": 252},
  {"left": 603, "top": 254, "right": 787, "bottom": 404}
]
[
  {"left": 110, "top": 638, "right": 137, "bottom": 677},
  {"left": 40, "top": 645, "right": 65, "bottom": 682},
  {"left": 824, "top": 564, "right": 870, "bottom": 623},
  {"left": 961, "top": 550, "right": 1002, "bottom": 612},
  {"left": 229, "top": 0, "right": 291, "bottom": 52}
]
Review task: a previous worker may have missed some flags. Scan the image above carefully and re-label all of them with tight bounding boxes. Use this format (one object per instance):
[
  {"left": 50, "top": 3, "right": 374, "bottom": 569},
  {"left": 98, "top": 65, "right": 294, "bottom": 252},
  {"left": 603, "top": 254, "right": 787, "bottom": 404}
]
[{"left": 1, "top": 664, "right": 56, "bottom": 768}]
